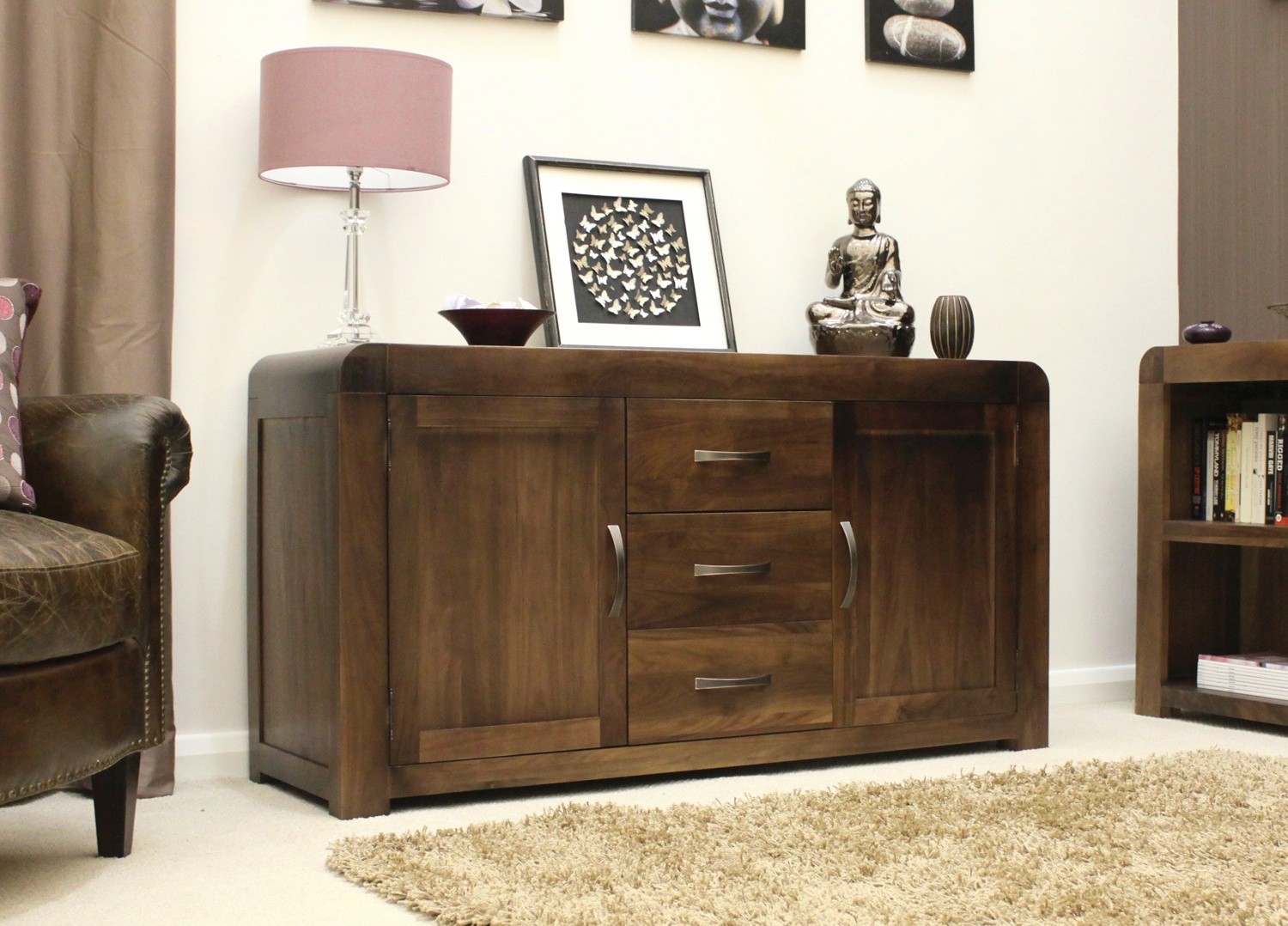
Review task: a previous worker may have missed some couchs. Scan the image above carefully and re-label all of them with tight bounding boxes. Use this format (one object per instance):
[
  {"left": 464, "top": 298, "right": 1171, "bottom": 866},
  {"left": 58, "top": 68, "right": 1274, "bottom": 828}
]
[{"left": 0, "top": 394, "right": 193, "bottom": 858}]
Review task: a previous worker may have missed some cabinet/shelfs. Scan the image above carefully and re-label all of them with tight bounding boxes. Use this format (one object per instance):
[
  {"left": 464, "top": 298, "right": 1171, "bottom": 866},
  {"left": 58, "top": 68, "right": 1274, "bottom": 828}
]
[
  {"left": 246, "top": 343, "right": 1049, "bottom": 819},
  {"left": 1136, "top": 340, "right": 1288, "bottom": 725}
]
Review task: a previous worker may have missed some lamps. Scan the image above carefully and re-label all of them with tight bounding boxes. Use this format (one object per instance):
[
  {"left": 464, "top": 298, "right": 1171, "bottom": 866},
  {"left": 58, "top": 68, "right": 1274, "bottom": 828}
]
[{"left": 257, "top": 47, "right": 452, "bottom": 347}]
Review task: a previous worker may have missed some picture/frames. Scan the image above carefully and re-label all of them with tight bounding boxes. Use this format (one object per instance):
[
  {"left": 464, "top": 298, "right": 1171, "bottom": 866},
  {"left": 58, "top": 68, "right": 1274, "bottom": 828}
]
[{"left": 522, "top": 154, "right": 737, "bottom": 352}]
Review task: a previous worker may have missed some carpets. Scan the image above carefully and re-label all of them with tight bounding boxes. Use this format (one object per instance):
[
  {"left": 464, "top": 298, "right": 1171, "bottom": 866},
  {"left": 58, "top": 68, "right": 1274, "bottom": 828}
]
[{"left": 326, "top": 745, "right": 1288, "bottom": 926}]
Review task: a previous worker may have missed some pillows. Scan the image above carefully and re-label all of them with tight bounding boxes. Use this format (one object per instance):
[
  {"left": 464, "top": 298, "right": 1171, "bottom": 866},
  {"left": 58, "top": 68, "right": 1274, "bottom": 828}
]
[{"left": 0, "top": 277, "right": 43, "bottom": 512}]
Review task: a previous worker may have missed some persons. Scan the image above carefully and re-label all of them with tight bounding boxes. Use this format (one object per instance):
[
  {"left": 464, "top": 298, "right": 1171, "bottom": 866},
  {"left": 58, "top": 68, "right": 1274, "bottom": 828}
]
[
  {"left": 807, "top": 177, "right": 914, "bottom": 325},
  {"left": 658, "top": 0, "right": 784, "bottom": 46}
]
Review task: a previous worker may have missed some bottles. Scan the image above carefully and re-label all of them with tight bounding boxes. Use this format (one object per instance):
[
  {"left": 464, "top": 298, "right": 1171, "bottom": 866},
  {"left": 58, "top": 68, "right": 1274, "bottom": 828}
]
[
  {"left": 1183, "top": 321, "right": 1232, "bottom": 344},
  {"left": 930, "top": 296, "right": 974, "bottom": 360}
]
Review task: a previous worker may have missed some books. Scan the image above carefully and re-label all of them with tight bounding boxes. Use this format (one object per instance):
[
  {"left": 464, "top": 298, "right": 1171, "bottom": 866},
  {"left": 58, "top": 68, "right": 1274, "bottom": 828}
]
[
  {"left": 1197, "top": 654, "right": 1288, "bottom": 700},
  {"left": 1190, "top": 412, "right": 1288, "bottom": 525}
]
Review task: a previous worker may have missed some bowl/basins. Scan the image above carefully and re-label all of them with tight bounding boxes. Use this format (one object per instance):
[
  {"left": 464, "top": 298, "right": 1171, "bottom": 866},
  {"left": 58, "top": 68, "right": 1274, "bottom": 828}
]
[{"left": 438, "top": 309, "right": 556, "bottom": 346}]
